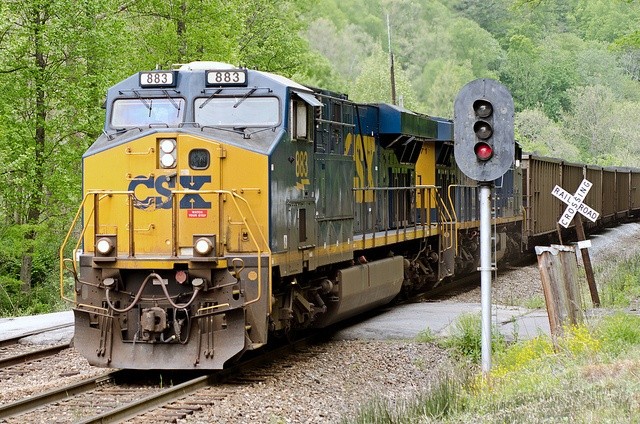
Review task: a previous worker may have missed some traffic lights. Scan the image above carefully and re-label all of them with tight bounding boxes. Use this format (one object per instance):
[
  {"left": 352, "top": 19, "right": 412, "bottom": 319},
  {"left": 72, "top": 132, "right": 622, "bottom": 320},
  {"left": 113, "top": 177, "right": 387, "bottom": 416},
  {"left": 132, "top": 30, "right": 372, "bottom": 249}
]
[{"left": 454, "top": 77, "right": 514, "bottom": 181}]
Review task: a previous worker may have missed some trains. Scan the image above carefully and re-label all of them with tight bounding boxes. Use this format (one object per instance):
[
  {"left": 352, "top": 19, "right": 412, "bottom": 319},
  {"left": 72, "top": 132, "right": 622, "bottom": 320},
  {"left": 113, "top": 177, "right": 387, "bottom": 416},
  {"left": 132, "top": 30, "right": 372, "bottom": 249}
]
[{"left": 59, "top": 61, "right": 640, "bottom": 383}]
